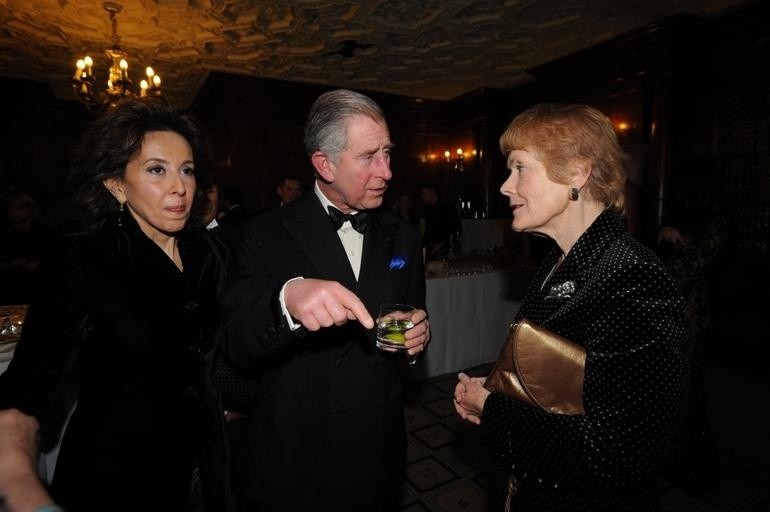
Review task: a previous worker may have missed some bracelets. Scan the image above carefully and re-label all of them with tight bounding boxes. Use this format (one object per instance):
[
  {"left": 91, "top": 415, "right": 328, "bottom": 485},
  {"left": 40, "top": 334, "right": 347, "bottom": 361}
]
[{"left": 37, "top": 505, "right": 61, "bottom": 512}]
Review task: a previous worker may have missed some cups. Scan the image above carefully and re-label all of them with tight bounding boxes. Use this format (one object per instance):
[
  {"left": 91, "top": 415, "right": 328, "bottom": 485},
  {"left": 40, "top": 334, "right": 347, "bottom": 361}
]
[{"left": 376, "top": 302, "right": 417, "bottom": 353}]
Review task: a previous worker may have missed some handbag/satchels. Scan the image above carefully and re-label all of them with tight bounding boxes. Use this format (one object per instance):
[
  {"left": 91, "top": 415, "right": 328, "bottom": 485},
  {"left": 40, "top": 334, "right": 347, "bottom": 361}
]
[{"left": 482, "top": 316, "right": 588, "bottom": 419}]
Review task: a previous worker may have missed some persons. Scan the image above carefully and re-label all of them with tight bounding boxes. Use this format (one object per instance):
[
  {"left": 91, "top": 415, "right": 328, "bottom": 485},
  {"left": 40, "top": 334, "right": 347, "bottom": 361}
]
[
  {"left": 0, "top": 98, "right": 232, "bottom": 512},
  {"left": 656, "top": 215, "right": 688, "bottom": 264},
  {"left": 212, "top": 86, "right": 432, "bottom": 511},
  {"left": 192, "top": 173, "right": 463, "bottom": 263},
  {"left": 451, "top": 101, "right": 714, "bottom": 512}
]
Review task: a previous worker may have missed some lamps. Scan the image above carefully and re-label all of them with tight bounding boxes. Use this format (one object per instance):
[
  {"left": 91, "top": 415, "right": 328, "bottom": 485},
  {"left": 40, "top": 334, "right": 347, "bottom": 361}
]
[{"left": 71, "top": 0, "right": 163, "bottom": 114}]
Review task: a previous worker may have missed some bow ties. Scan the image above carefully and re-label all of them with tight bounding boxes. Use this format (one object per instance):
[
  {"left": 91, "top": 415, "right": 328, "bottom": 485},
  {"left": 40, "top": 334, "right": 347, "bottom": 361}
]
[{"left": 327, "top": 205, "right": 370, "bottom": 234}]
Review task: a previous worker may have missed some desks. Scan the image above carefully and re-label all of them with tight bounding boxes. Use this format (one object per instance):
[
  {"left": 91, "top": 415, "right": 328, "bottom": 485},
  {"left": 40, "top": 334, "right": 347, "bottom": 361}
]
[{"left": 423, "top": 259, "right": 519, "bottom": 377}]
